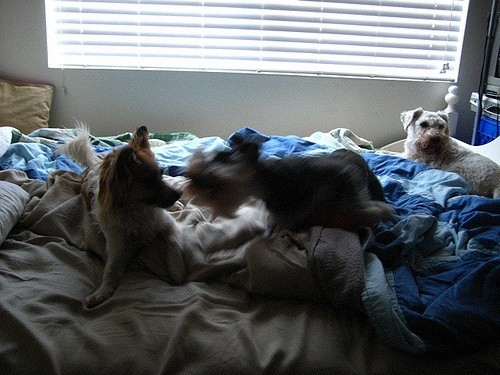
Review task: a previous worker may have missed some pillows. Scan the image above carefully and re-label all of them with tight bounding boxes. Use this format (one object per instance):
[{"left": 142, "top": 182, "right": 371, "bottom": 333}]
[
  {"left": 0, "top": 79, "right": 54, "bottom": 134},
  {"left": 0, "top": 180, "right": 30, "bottom": 245}
]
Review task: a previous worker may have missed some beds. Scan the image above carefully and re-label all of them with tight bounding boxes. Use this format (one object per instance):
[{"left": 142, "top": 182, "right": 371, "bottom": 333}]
[{"left": 0, "top": 129, "right": 499, "bottom": 374}]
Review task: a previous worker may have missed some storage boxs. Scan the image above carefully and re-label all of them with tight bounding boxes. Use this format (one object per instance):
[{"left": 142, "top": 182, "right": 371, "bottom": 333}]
[{"left": 479, "top": 116, "right": 500, "bottom": 144}]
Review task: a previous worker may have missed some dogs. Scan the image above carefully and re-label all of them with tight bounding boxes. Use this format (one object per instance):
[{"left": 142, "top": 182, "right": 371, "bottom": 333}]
[
  {"left": 375, "top": 107, "right": 500, "bottom": 200},
  {"left": 187, "top": 140, "right": 397, "bottom": 232},
  {"left": 54, "top": 116, "right": 187, "bottom": 308}
]
